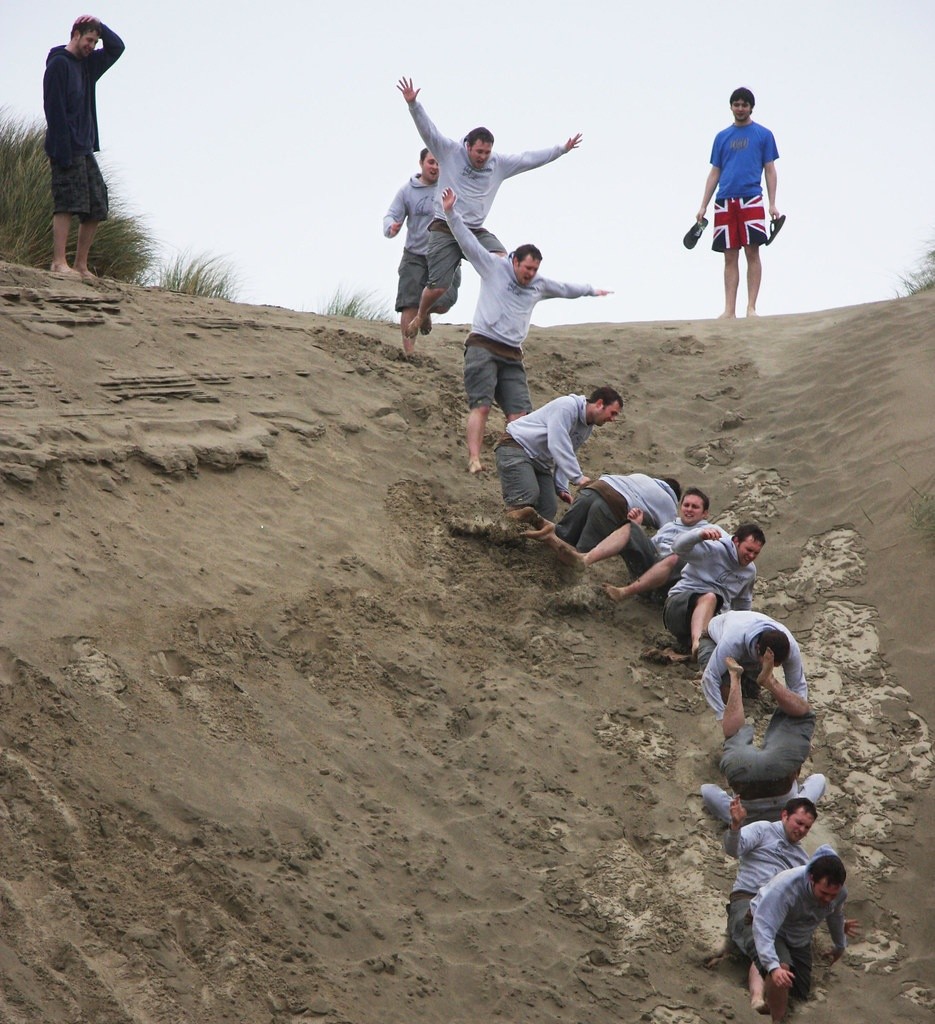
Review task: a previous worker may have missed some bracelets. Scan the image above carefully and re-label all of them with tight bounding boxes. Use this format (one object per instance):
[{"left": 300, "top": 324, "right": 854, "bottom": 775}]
[{"left": 843, "top": 918, "right": 847, "bottom": 923}]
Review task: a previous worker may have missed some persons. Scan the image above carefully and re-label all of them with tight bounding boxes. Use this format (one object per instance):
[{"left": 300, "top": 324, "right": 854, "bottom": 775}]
[
  {"left": 494, "top": 387, "right": 624, "bottom": 531},
  {"left": 437, "top": 186, "right": 614, "bottom": 474},
  {"left": 724, "top": 792, "right": 819, "bottom": 1010},
  {"left": 557, "top": 485, "right": 710, "bottom": 608},
  {"left": 741, "top": 842, "right": 849, "bottom": 1024},
  {"left": 639, "top": 522, "right": 765, "bottom": 666},
  {"left": 505, "top": 471, "right": 682, "bottom": 559},
  {"left": 696, "top": 610, "right": 808, "bottom": 722},
  {"left": 382, "top": 147, "right": 461, "bottom": 358},
  {"left": 42, "top": 15, "right": 125, "bottom": 277},
  {"left": 695, "top": 87, "right": 779, "bottom": 320},
  {"left": 700, "top": 648, "right": 827, "bottom": 827},
  {"left": 397, "top": 75, "right": 584, "bottom": 338}
]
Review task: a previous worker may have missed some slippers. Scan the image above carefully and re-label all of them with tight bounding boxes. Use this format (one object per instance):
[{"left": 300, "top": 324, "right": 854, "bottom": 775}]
[
  {"left": 684, "top": 217, "right": 708, "bottom": 249},
  {"left": 766, "top": 215, "right": 786, "bottom": 245}
]
